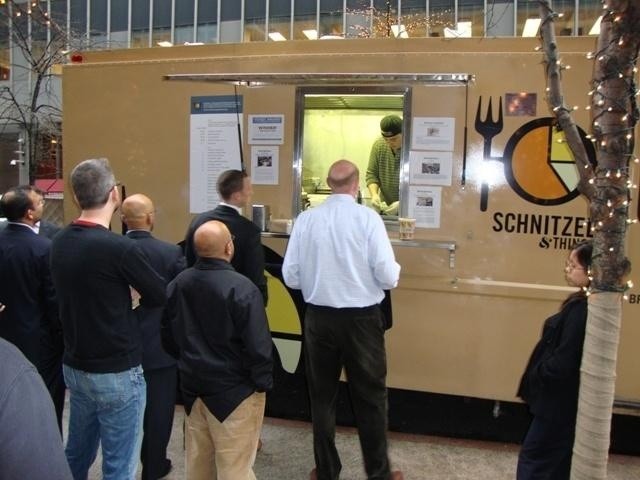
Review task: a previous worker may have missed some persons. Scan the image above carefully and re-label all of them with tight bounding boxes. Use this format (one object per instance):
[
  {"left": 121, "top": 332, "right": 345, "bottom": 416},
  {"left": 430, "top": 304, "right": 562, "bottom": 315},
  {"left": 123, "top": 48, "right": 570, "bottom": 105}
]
[
  {"left": 0, "top": 183, "right": 66, "bottom": 448},
  {"left": 181, "top": 168, "right": 270, "bottom": 455},
  {"left": 119, "top": 193, "right": 191, "bottom": 478},
  {"left": 0, "top": 335, "right": 74, "bottom": 479},
  {"left": 0, "top": 187, "right": 62, "bottom": 242},
  {"left": 49, "top": 157, "right": 169, "bottom": 479},
  {"left": 514, "top": 237, "right": 595, "bottom": 479},
  {"left": 258, "top": 155, "right": 272, "bottom": 167},
  {"left": 364, "top": 115, "right": 404, "bottom": 218},
  {"left": 280, "top": 157, "right": 404, "bottom": 480},
  {"left": 158, "top": 219, "right": 279, "bottom": 479}
]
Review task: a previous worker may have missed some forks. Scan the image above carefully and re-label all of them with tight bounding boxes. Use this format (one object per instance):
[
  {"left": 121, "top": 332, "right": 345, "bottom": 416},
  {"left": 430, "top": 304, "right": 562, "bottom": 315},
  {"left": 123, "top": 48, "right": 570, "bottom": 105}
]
[{"left": 474, "top": 94, "right": 505, "bottom": 213}]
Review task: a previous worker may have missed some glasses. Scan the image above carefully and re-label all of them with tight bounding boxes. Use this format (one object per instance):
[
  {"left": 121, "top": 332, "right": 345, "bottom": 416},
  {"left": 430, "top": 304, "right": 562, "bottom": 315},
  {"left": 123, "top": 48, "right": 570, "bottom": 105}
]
[
  {"left": 567, "top": 260, "right": 586, "bottom": 272},
  {"left": 110, "top": 182, "right": 122, "bottom": 193},
  {"left": 229, "top": 234, "right": 236, "bottom": 243}
]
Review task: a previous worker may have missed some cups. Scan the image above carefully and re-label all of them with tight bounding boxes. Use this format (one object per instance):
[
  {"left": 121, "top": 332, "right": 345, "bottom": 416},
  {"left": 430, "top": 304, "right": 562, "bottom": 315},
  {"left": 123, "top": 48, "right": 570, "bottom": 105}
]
[
  {"left": 397, "top": 216, "right": 417, "bottom": 241},
  {"left": 251, "top": 204, "right": 273, "bottom": 232}
]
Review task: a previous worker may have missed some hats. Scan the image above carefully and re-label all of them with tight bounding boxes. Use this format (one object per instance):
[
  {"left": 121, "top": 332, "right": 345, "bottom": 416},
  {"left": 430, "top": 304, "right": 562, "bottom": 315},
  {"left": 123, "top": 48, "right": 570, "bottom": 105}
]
[{"left": 380, "top": 115, "right": 403, "bottom": 137}]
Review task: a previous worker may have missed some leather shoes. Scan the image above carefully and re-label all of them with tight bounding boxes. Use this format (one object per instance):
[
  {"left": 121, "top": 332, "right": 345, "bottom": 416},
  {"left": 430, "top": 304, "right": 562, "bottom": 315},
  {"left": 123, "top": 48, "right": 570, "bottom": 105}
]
[
  {"left": 311, "top": 468, "right": 318, "bottom": 479},
  {"left": 257, "top": 441, "right": 262, "bottom": 451},
  {"left": 391, "top": 471, "right": 403, "bottom": 479},
  {"left": 141, "top": 460, "right": 171, "bottom": 478}
]
